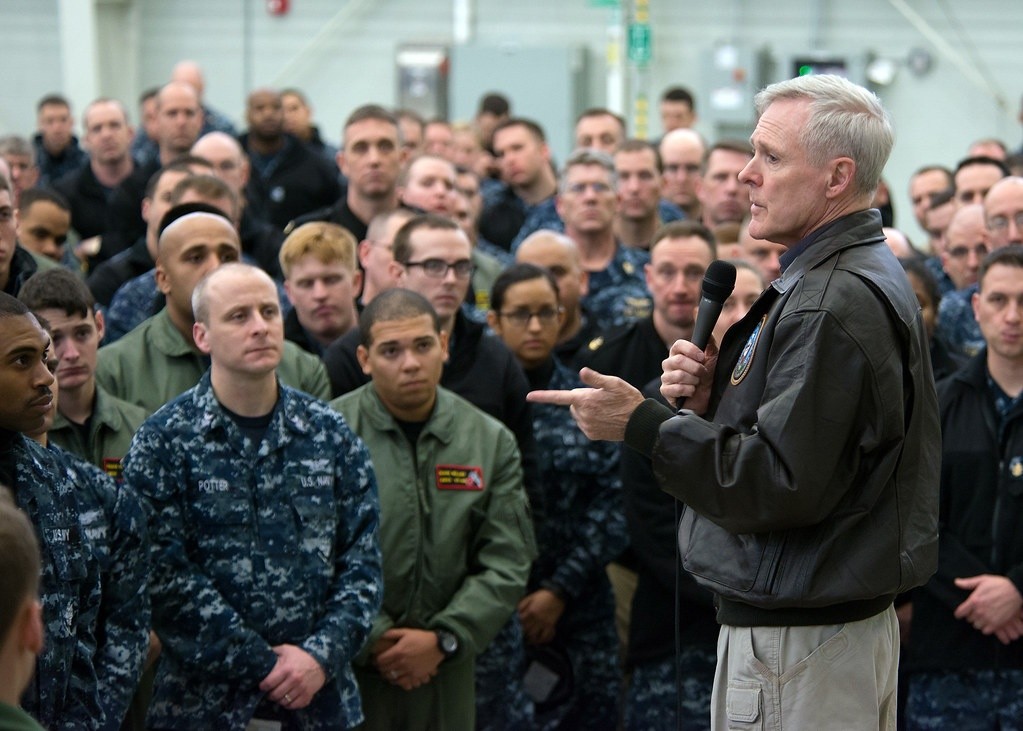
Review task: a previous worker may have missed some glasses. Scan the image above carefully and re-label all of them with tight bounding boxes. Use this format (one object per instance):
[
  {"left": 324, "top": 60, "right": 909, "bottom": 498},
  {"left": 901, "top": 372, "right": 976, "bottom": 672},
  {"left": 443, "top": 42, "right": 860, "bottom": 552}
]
[
  {"left": 498, "top": 308, "right": 562, "bottom": 328},
  {"left": 404, "top": 258, "right": 475, "bottom": 281}
]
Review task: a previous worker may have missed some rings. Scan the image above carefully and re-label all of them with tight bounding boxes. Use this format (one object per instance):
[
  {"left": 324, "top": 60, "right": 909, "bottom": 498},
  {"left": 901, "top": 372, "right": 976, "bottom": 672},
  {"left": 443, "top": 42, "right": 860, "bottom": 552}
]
[{"left": 285, "top": 694, "right": 292, "bottom": 703}]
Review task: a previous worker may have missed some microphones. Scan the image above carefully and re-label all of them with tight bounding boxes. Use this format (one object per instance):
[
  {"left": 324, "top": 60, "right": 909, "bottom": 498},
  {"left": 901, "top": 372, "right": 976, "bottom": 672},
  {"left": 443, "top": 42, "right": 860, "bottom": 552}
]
[{"left": 677, "top": 260, "right": 736, "bottom": 407}]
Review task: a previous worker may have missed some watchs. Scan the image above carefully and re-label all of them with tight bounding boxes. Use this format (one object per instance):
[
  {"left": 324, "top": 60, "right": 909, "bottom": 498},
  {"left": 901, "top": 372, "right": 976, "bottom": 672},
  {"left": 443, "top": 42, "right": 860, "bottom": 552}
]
[{"left": 434, "top": 626, "right": 458, "bottom": 656}]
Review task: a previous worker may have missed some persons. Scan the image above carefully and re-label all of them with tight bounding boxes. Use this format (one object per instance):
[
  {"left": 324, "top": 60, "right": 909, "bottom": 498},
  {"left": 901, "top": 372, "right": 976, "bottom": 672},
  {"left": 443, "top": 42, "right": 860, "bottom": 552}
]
[
  {"left": 0, "top": 61, "right": 1023, "bottom": 731},
  {"left": 526, "top": 75, "right": 943, "bottom": 731}
]
